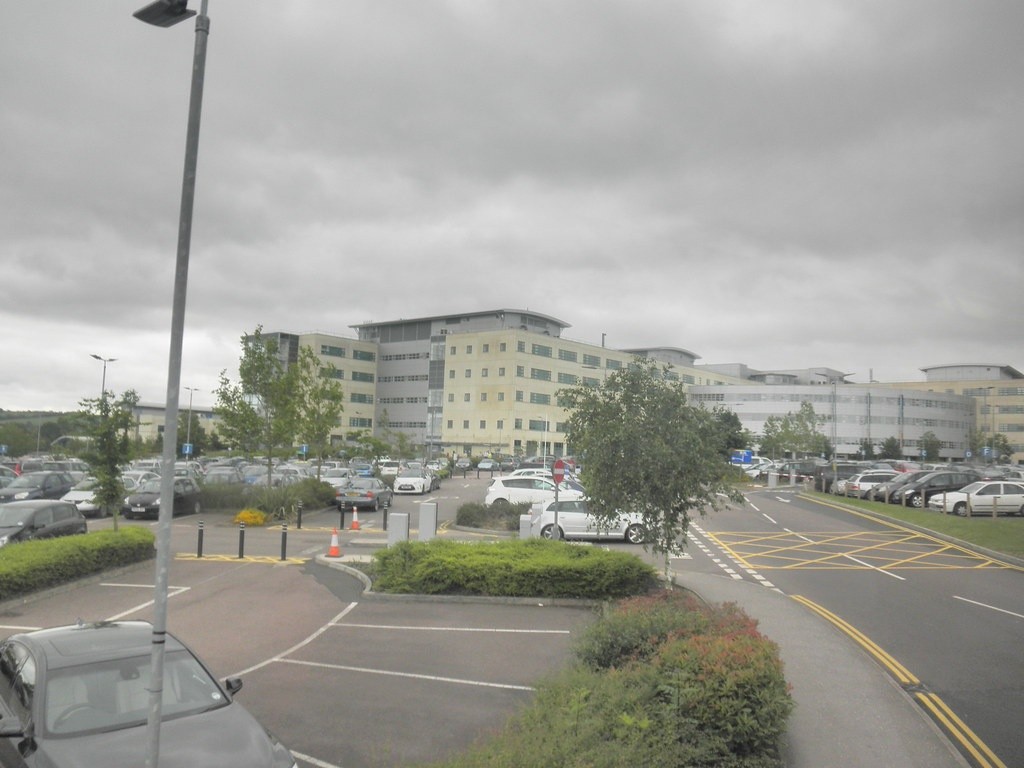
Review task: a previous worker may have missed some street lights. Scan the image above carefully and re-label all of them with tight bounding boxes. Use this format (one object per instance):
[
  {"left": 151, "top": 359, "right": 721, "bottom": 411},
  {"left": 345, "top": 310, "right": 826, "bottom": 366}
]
[
  {"left": 816, "top": 372, "right": 855, "bottom": 494},
  {"left": 132, "top": 1, "right": 211, "bottom": 767},
  {"left": 185, "top": 387, "right": 200, "bottom": 470},
  {"left": 91, "top": 354, "right": 118, "bottom": 437}
]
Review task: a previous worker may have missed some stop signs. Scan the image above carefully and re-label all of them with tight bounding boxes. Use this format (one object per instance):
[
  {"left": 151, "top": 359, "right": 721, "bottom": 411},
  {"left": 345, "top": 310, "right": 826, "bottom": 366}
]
[{"left": 552, "top": 459, "right": 564, "bottom": 483}]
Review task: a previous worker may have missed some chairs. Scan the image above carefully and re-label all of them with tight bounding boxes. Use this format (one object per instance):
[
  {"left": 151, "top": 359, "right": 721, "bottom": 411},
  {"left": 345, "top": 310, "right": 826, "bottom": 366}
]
[
  {"left": 114, "top": 662, "right": 181, "bottom": 712},
  {"left": 46, "top": 675, "right": 88, "bottom": 732}
]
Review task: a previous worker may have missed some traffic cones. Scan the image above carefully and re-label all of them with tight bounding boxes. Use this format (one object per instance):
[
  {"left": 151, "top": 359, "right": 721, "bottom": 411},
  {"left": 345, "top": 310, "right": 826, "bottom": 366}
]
[
  {"left": 348, "top": 506, "right": 362, "bottom": 530},
  {"left": 325, "top": 528, "right": 343, "bottom": 557}
]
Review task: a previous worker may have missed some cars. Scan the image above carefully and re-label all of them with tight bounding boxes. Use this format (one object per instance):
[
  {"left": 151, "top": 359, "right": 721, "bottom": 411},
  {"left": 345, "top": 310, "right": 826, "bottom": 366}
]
[
  {"left": 0, "top": 435, "right": 454, "bottom": 518},
  {"left": 928, "top": 480, "right": 1024, "bottom": 518},
  {"left": 729, "top": 456, "right": 1024, "bottom": 503},
  {"left": 484, "top": 474, "right": 583, "bottom": 509},
  {"left": 0, "top": 499, "right": 87, "bottom": 547},
  {"left": 891, "top": 470, "right": 982, "bottom": 509},
  {"left": 455, "top": 457, "right": 474, "bottom": 471},
  {"left": 501, "top": 458, "right": 517, "bottom": 471},
  {"left": 0, "top": 619, "right": 299, "bottom": 768},
  {"left": 393, "top": 468, "right": 432, "bottom": 495},
  {"left": 123, "top": 475, "right": 206, "bottom": 522},
  {"left": 336, "top": 477, "right": 394, "bottom": 512},
  {"left": 478, "top": 458, "right": 498, "bottom": 471},
  {"left": 525, "top": 492, "right": 656, "bottom": 545}
]
